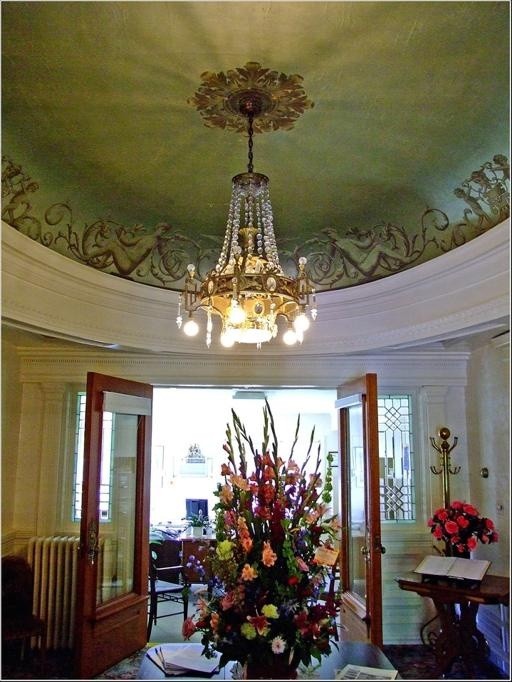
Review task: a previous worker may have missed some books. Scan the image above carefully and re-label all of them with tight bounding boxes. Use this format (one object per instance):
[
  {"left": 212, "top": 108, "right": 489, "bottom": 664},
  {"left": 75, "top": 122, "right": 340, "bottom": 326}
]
[
  {"left": 413, "top": 553, "right": 492, "bottom": 583},
  {"left": 146, "top": 642, "right": 225, "bottom": 676},
  {"left": 337, "top": 664, "right": 398, "bottom": 680}
]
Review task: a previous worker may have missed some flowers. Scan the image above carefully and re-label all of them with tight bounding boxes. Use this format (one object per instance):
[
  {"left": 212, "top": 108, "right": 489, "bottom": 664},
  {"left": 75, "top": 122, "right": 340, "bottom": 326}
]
[
  {"left": 180, "top": 396, "right": 346, "bottom": 669},
  {"left": 428, "top": 501, "right": 499, "bottom": 559}
]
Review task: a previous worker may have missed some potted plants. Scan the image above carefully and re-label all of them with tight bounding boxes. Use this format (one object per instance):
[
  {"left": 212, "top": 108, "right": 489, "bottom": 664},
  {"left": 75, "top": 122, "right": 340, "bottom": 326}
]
[{"left": 188, "top": 514, "right": 208, "bottom": 536}]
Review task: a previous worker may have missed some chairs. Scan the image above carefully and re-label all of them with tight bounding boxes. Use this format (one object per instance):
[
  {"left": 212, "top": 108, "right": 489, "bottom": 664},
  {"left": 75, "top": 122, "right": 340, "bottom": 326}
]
[{"left": 148, "top": 546, "right": 188, "bottom": 642}]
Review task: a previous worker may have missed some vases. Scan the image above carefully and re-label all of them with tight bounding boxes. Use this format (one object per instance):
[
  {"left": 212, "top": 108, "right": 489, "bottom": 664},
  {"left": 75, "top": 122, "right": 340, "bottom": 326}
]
[{"left": 241, "top": 652, "right": 297, "bottom": 678}]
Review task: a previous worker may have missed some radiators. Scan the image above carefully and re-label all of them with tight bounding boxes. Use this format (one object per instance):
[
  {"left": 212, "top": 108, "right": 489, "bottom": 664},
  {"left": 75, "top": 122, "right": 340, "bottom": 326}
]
[{"left": 30, "top": 534, "right": 112, "bottom": 652}]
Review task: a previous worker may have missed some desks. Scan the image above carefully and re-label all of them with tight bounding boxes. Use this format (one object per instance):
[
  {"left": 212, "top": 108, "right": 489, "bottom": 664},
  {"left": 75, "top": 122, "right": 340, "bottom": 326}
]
[
  {"left": 137, "top": 641, "right": 403, "bottom": 678},
  {"left": 396, "top": 571, "right": 510, "bottom": 679},
  {"left": 181, "top": 526, "right": 221, "bottom": 584}
]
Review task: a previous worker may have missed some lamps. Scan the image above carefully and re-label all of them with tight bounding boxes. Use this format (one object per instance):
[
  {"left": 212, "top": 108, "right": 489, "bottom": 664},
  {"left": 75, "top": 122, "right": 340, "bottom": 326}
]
[{"left": 176, "top": 64, "right": 317, "bottom": 347}]
